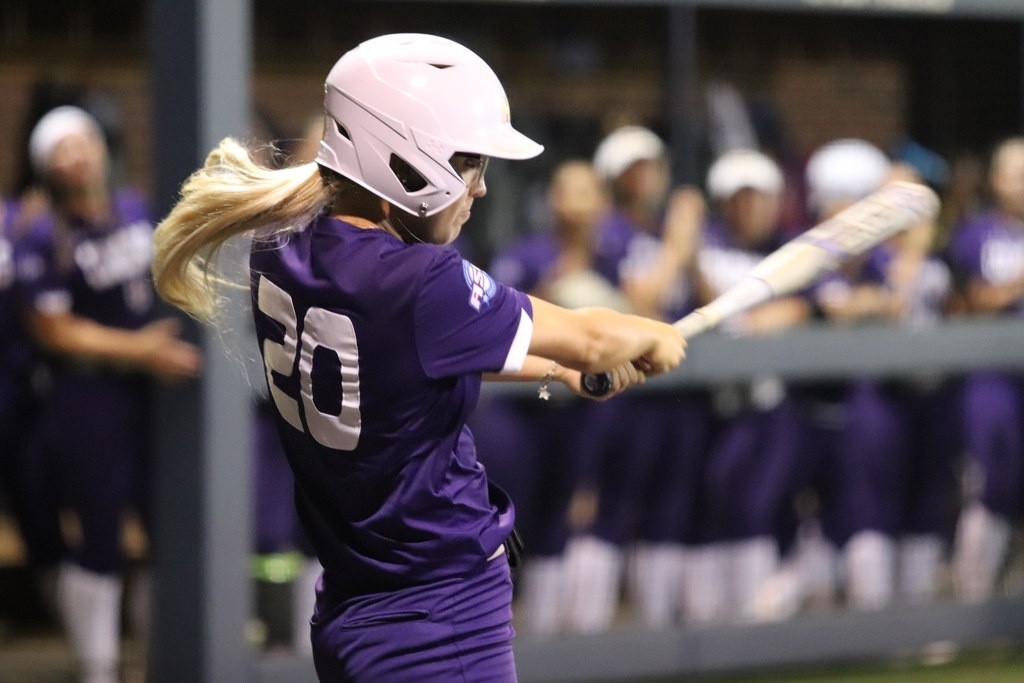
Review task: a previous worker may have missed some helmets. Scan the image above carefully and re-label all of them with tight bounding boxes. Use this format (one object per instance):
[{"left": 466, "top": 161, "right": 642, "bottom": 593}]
[
  {"left": 594, "top": 124, "right": 663, "bottom": 180},
  {"left": 807, "top": 138, "right": 888, "bottom": 211},
  {"left": 315, "top": 34, "right": 545, "bottom": 218},
  {"left": 708, "top": 148, "right": 782, "bottom": 199}
]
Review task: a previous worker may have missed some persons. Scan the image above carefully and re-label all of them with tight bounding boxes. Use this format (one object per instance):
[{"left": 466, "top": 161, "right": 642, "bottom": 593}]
[
  {"left": 0, "top": 106, "right": 1024, "bottom": 683},
  {"left": 153, "top": 34, "right": 688, "bottom": 683}
]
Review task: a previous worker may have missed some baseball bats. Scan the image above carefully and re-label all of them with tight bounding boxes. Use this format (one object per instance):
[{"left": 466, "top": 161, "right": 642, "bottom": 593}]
[{"left": 579, "top": 182, "right": 941, "bottom": 398}]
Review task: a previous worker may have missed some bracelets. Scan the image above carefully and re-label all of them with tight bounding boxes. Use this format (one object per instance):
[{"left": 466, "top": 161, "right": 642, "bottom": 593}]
[{"left": 537, "top": 360, "right": 556, "bottom": 401}]
